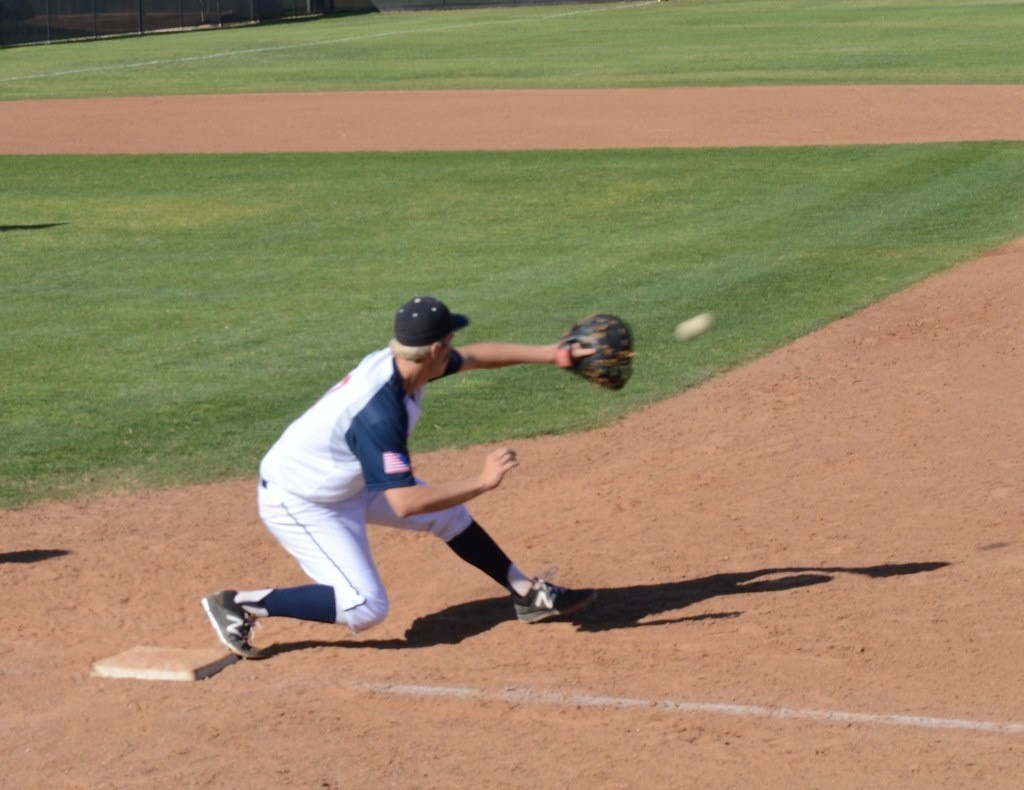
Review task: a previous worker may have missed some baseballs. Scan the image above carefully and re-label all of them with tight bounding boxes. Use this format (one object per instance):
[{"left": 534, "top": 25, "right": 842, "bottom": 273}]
[{"left": 676, "top": 312, "right": 713, "bottom": 342}]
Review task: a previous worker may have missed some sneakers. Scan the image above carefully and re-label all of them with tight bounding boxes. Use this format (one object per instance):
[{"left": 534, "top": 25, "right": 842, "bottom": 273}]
[
  {"left": 512, "top": 566, "right": 597, "bottom": 624},
  {"left": 202, "top": 590, "right": 263, "bottom": 658}
]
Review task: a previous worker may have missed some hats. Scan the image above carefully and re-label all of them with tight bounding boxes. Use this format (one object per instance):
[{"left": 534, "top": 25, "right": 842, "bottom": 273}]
[{"left": 394, "top": 295, "right": 470, "bottom": 346}]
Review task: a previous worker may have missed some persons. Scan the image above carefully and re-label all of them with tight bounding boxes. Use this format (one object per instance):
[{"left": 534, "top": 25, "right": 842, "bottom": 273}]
[{"left": 200, "top": 296, "right": 634, "bottom": 660}]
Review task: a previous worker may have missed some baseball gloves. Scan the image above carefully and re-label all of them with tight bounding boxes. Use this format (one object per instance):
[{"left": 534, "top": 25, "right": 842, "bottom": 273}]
[{"left": 563, "top": 312, "right": 636, "bottom": 391}]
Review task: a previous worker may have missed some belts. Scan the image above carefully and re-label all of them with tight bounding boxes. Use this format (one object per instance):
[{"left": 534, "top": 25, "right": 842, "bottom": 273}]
[{"left": 262, "top": 480, "right": 267, "bottom": 487}]
